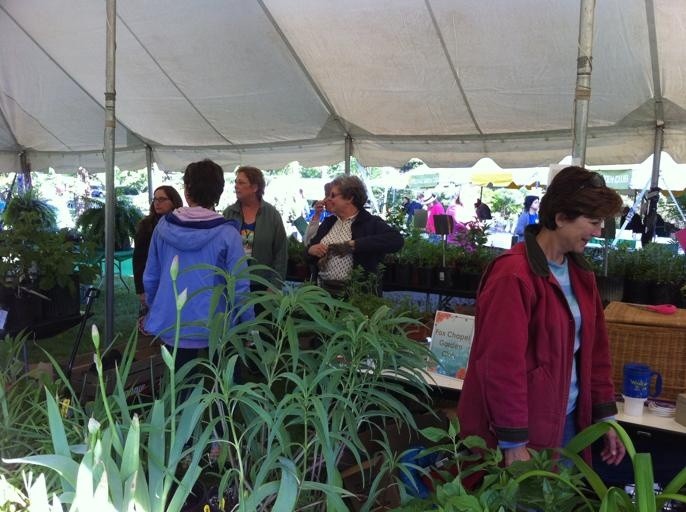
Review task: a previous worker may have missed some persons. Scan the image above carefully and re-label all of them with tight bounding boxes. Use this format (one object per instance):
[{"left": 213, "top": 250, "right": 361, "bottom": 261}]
[
  {"left": 303, "top": 182, "right": 336, "bottom": 284},
  {"left": 644, "top": 208, "right": 665, "bottom": 237},
  {"left": 302, "top": 175, "right": 404, "bottom": 301},
  {"left": 401, "top": 192, "right": 492, "bottom": 233},
  {"left": 620, "top": 207, "right": 641, "bottom": 233},
  {"left": 513, "top": 195, "right": 540, "bottom": 243},
  {"left": 132, "top": 185, "right": 183, "bottom": 307},
  {"left": 421, "top": 165, "right": 625, "bottom": 492},
  {"left": 142, "top": 159, "right": 259, "bottom": 459},
  {"left": 221, "top": 165, "right": 287, "bottom": 374}
]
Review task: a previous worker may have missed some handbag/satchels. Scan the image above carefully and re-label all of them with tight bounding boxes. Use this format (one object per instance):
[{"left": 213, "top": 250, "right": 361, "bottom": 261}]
[{"left": 139, "top": 316, "right": 153, "bottom": 337}]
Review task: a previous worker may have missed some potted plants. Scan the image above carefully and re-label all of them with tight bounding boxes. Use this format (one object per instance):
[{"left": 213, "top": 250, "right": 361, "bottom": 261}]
[
  {"left": 0, "top": 183, "right": 145, "bottom": 327},
  {"left": 286, "top": 227, "right": 686, "bottom": 308}
]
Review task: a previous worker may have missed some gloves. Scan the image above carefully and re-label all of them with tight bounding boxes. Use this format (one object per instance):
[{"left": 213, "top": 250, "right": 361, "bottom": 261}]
[
  {"left": 317, "top": 254, "right": 332, "bottom": 271},
  {"left": 329, "top": 241, "right": 352, "bottom": 257}
]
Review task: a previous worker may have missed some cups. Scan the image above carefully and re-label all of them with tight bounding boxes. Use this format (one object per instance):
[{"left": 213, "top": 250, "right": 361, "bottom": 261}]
[{"left": 622, "top": 363, "right": 663, "bottom": 399}]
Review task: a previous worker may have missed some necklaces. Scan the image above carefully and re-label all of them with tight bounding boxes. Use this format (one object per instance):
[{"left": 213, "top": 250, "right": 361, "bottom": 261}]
[{"left": 240, "top": 204, "right": 256, "bottom": 246}]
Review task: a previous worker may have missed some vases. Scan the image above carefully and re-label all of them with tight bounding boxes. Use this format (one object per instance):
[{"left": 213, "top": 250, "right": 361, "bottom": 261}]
[{"left": 164, "top": 475, "right": 237, "bottom": 512}]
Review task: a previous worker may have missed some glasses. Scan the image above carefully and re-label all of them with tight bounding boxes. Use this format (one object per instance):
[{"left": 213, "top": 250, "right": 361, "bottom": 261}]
[
  {"left": 572, "top": 173, "right": 605, "bottom": 195},
  {"left": 332, "top": 192, "right": 342, "bottom": 198},
  {"left": 153, "top": 197, "right": 171, "bottom": 202}
]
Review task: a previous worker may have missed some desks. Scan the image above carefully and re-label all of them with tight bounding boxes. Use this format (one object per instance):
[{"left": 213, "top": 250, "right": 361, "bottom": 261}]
[{"left": 357, "top": 366, "right": 686, "bottom": 512}]
